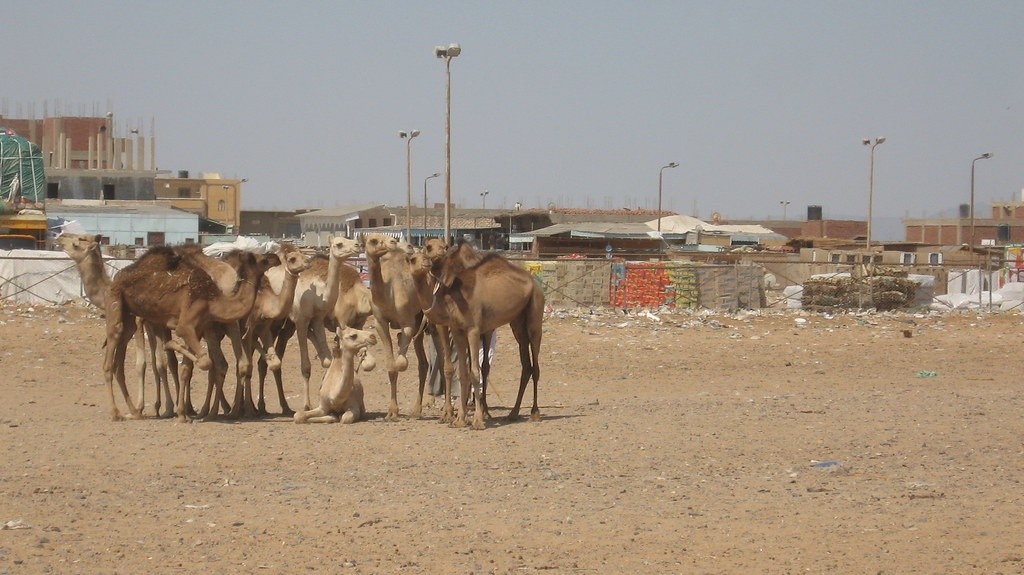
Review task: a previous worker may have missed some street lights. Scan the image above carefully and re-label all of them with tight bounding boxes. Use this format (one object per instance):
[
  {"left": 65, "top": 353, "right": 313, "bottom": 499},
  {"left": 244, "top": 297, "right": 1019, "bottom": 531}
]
[
  {"left": 479, "top": 191, "right": 490, "bottom": 209},
  {"left": 223, "top": 185, "right": 229, "bottom": 225},
  {"left": 780, "top": 200, "right": 791, "bottom": 219},
  {"left": 436, "top": 43, "right": 462, "bottom": 243},
  {"left": 424, "top": 173, "right": 441, "bottom": 228},
  {"left": 234, "top": 178, "right": 248, "bottom": 225},
  {"left": 399, "top": 129, "right": 420, "bottom": 243},
  {"left": 970, "top": 152, "right": 993, "bottom": 260},
  {"left": 658, "top": 162, "right": 680, "bottom": 231},
  {"left": 862, "top": 136, "right": 886, "bottom": 250}
]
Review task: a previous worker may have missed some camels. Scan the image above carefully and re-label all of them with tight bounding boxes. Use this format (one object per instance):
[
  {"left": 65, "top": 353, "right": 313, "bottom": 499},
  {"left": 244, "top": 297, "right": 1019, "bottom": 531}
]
[{"left": 52, "top": 228, "right": 545, "bottom": 430}]
